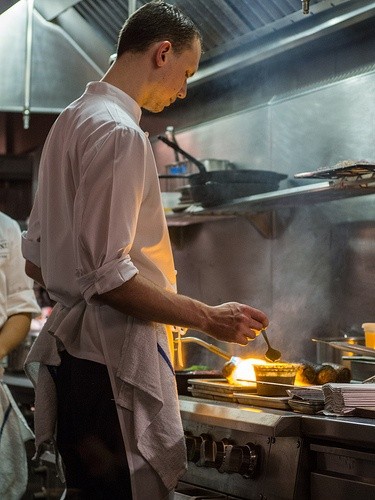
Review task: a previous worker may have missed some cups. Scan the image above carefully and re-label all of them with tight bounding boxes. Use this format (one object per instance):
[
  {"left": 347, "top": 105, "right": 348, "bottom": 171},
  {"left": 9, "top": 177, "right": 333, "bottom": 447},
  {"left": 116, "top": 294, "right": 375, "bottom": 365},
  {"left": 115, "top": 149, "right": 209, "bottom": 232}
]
[{"left": 362, "top": 323, "right": 375, "bottom": 348}]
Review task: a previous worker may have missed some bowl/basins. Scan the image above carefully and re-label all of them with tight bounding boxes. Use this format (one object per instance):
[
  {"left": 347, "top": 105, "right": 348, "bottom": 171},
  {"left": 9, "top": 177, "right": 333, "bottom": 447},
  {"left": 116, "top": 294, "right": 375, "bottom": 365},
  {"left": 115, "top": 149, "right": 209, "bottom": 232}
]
[{"left": 252, "top": 363, "right": 298, "bottom": 395}]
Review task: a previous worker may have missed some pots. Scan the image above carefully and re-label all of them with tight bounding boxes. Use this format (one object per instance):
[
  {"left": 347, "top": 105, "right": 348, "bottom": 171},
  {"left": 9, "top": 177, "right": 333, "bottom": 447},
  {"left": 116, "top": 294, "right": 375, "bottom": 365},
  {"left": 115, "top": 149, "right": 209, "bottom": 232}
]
[
  {"left": 189, "top": 158, "right": 237, "bottom": 174},
  {"left": 157, "top": 135, "right": 288, "bottom": 208}
]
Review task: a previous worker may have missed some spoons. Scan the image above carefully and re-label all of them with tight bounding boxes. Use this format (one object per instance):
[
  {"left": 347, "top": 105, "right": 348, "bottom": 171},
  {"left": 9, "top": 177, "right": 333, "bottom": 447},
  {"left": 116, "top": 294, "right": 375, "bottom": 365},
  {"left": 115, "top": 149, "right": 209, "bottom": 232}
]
[{"left": 262, "top": 330, "right": 281, "bottom": 361}]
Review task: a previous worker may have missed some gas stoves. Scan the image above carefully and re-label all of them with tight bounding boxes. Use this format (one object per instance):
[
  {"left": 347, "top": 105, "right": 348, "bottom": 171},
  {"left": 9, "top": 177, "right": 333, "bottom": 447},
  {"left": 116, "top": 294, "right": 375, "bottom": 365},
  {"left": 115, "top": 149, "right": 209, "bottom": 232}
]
[{"left": 173, "top": 392, "right": 310, "bottom": 500}]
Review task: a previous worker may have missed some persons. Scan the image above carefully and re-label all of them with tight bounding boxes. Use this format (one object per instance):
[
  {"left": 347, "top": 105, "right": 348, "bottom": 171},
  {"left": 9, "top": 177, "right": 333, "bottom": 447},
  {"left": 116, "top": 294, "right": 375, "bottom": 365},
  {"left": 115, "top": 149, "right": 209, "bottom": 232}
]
[
  {"left": 0, "top": 211, "right": 42, "bottom": 378},
  {"left": 22, "top": 0, "right": 270, "bottom": 500}
]
